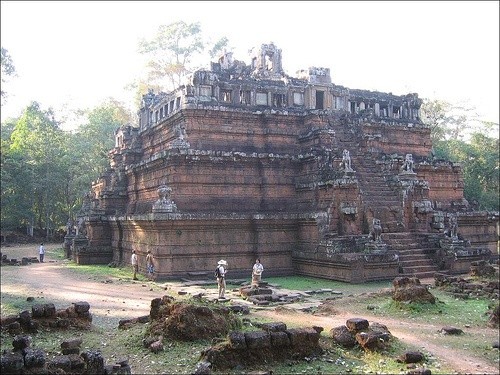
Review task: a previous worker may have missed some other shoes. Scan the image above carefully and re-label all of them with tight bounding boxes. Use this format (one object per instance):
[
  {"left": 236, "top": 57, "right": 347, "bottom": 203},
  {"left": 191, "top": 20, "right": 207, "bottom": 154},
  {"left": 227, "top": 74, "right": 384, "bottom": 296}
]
[{"left": 133, "top": 278, "right": 138, "bottom": 280}]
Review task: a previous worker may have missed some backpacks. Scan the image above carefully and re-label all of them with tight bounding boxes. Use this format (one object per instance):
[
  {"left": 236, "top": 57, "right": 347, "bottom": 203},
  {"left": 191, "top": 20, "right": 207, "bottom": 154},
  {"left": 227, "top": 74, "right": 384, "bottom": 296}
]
[{"left": 214, "top": 266, "right": 225, "bottom": 278}]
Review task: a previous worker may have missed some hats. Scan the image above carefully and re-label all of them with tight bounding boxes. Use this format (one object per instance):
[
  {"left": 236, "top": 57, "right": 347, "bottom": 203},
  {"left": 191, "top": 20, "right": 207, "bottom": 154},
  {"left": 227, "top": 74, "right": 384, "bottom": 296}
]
[{"left": 217, "top": 259, "right": 227, "bottom": 264}]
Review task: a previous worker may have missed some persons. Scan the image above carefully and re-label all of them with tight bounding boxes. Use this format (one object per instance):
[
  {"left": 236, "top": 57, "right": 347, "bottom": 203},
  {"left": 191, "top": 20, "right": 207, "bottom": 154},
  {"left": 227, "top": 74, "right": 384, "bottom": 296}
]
[
  {"left": 132, "top": 250, "right": 138, "bottom": 280},
  {"left": 39, "top": 243, "right": 45, "bottom": 262},
  {"left": 253, "top": 259, "right": 264, "bottom": 281},
  {"left": 146, "top": 251, "right": 154, "bottom": 281},
  {"left": 216, "top": 260, "right": 228, "bottom": 298}
]
